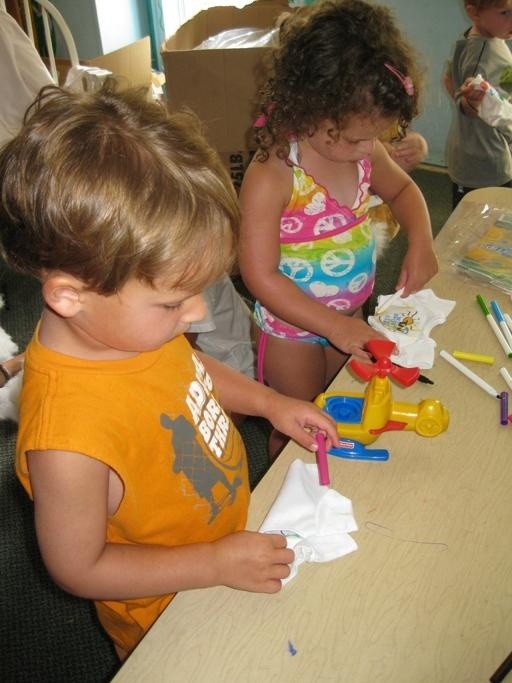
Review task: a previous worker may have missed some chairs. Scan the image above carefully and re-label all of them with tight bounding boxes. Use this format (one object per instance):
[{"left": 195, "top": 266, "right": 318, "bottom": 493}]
[{"left": 0, "top": 0, "right": 83, "bottom": 97}]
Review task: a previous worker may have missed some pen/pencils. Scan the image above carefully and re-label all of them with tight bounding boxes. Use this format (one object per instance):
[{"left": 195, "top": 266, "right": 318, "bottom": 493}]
[
  {"left": 439, "top": 294, "right": 512, "bottom": 425},
  {"left": 314, "top": 433, "right": 330, "bottom": 486},
  {"left": 362, "top": 349, "right": 434, "bottom": 384}
]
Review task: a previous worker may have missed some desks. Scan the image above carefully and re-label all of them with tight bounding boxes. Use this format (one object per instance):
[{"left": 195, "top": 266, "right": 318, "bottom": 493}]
[{"left": 108, "top": 187, "right": 512, "bottom": 683}]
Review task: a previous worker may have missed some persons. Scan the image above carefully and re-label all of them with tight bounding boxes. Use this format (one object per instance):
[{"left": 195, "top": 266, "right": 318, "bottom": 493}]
[
  {"left": 443, "top": 0, "right": 512, "bottom": 209},
  {"left": 0, "top": 74, "right": 338, "bottom": 668},
  {"left": 0, "top": 350, "right": 26, "bottom": 392},
  {"left": 176, "top": 260, "right": 259, "bottom": 427},
  {"left": 235, "top": 0, "right": 443, "bottom": 461},
  {"left": 365, "top": 130, "right": 431, "bottom": 253}
]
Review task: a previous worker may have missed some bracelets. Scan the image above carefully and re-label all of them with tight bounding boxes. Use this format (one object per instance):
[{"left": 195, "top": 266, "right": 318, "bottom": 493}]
[
  {"left": 0, "top": 363, "right": 12, "bottom": 380},
  {"left": 464, "top": 97, "right": 478, "bottom": 113}
]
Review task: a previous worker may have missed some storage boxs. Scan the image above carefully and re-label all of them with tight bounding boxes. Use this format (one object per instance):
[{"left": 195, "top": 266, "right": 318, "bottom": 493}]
[
  {"left": 159, "top": 0, "right": 316, "bottom": 197},
  {"left": 44, "top": 35, "right": 153, "bottom": 104}
]
